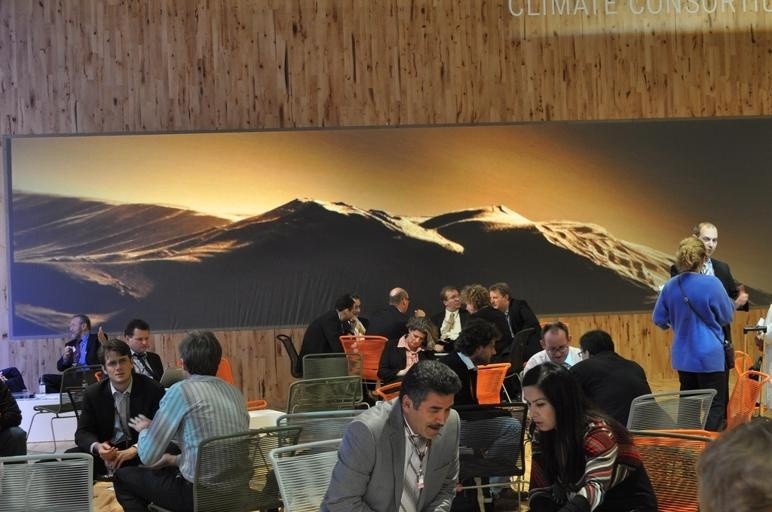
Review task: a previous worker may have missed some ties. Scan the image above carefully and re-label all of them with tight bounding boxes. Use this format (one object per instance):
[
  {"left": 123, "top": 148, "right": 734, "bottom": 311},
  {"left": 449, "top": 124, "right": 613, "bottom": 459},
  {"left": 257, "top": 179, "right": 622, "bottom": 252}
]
[
  {"left": 72, "top": 339, "right": 82, "bottom": 367},
  {"left": 441, "top": 313, "right": 455, "bottom": 340},
  {"left": 133, "top": 354, "right": 156, "bottom": 379}
]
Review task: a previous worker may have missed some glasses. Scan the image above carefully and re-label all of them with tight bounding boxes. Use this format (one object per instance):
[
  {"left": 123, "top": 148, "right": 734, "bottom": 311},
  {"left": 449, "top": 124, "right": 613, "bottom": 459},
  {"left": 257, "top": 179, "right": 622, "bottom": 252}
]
[
  {"left": 578, "top": 350, "right": 586, "bottom": 358},
  {"left": 546, "top": 346, "right": 568, "bottom": 353}
]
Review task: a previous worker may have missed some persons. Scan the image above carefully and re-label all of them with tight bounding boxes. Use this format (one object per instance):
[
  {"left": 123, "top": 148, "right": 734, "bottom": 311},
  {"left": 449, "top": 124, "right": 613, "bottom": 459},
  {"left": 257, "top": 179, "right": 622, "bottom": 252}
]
[
  {"left": 691, "top": 417, "right": 772, "bottom": 512},
  {"left": 670, "top": 220, "right": 750, "bottom": 431},
  {"left": 436, "top": 317, "right": 531, "bottom": 501},
  {"left": 756, "top": 299, "right": 772, "bottom": 414},
  {"left": 522, "top": 361, "right": 660, "bottom": 512},
  {"left": 1, "top": 382, "right": 26, "bottom": 464},
  {"left": 651, "top": 235, "right": 734, "bottom": 433},
  {"left": 367, "top": 282, "right": 673, "bottom": 430},
  {"left": 296, "top": 285, "right": 657, "bottom": 431},
  {"left": 38, "top": 315, "right": 251, "bottom": 511},
  {"left": 320, "top": 360, "right": 463, "bottom": 511}
]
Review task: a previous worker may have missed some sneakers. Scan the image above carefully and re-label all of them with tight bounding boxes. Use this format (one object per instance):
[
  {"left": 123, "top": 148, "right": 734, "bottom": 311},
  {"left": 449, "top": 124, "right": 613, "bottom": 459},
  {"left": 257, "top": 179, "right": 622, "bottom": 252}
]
[{"left": 491, "top": 488, "right": 529, "bottom": 502}]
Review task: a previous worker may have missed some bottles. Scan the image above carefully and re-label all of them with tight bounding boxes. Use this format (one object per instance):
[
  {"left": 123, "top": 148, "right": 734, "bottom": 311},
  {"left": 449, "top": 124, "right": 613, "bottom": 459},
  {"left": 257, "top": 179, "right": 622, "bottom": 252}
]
[{"left": 39, "top": 374, "right": 47, "bottom": 396}]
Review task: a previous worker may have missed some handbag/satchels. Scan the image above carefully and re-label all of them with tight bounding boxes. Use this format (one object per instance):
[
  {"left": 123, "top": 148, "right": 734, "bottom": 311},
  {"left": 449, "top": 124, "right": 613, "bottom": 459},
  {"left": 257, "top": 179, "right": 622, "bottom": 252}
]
[{"left": 724, "top": 340, "right": 734, "bottom": 370}]
